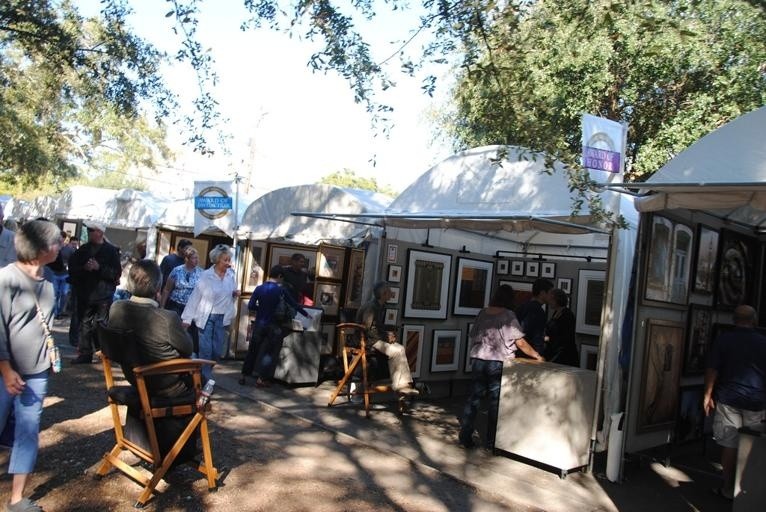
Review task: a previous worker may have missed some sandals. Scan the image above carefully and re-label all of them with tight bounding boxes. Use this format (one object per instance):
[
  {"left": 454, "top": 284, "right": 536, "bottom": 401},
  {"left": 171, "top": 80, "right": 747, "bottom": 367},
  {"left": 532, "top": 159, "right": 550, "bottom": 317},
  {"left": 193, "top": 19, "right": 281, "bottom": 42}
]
[
  {"left": 255, "top": 380, "right": 273, "bottom": 389},
  {"left": 239, "top": 375, "right": 245, "bottom": 385}
]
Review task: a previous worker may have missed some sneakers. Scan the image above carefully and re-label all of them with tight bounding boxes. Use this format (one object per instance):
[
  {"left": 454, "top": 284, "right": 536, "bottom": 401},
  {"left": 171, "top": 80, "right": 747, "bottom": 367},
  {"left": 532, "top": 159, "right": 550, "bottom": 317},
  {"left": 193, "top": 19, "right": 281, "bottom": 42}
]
[
  {"left": 398, "top": 387, "right": 420, "bottom": 397},
  {"left": 6, "top": 496, "right": 45, "bottom": 512}
]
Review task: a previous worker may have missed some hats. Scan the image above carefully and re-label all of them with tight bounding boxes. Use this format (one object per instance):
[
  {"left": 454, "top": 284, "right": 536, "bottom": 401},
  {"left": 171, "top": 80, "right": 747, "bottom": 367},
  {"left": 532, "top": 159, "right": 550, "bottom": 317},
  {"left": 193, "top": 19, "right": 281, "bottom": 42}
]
[{"left": 82, "top": 219, "right": 106, "bottom": 233}]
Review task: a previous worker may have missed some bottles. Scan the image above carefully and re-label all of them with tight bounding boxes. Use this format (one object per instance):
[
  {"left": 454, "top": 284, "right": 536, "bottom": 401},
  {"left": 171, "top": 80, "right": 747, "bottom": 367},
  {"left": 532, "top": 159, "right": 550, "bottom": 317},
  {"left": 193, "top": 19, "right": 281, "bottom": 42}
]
[{"left": 194, "top": 379, "right": 215, "bottom": 410}]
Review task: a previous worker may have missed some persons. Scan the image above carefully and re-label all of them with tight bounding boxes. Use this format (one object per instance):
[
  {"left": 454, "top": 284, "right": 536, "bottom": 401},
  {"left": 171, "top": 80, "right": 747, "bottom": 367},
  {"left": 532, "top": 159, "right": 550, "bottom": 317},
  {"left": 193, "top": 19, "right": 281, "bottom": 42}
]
[
  {"left": 355, "top": 281, "right": 420, "bottom": 398},
  {"left": 458, "top": 285, "right": 546, "bottom": 454},
  {"left": 703, "top": 304, "right": 766, "bottom": 500},
  {"left": 544, "top": 289, "right": 581, "bottom": 367},
  {"left": 513, "top": 278, "right": 554, "bottom": 358},
  {"left": 0, "top": 220, "right": 61, "bottom": 512},
  {"left": 107, "top": 260, "right": 208, "bottom": 468}
]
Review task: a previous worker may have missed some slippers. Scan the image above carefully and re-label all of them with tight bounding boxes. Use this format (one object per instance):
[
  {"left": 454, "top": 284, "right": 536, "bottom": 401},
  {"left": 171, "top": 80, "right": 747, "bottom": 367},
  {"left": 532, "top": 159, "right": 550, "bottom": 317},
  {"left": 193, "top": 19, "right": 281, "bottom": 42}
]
[{"left": 710, "top": 487, "right": 733, "bottom": 501}]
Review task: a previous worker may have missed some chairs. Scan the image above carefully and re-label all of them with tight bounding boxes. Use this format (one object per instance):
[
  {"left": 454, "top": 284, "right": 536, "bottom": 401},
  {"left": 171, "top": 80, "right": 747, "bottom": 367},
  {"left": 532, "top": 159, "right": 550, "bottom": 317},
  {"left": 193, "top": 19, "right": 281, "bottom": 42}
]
[
  {"left": 327, "top": 323, "right": 403, "bottom": 418},
  {"left": 85, "top": 316, "right": 223, "bottom": 509}
]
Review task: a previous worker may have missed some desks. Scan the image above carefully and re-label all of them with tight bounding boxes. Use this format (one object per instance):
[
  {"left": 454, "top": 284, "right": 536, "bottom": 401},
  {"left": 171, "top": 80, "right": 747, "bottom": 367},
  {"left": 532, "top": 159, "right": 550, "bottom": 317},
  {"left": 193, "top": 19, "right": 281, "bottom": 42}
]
[{"left": 495, "top": 357, "right": 598, "bottom": 480}]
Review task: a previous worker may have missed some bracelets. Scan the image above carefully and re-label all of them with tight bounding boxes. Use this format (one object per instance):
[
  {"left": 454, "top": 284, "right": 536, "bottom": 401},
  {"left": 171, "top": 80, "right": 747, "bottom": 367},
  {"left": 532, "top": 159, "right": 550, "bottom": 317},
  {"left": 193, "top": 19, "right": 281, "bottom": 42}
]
[{"left": 534, "top": 353, "right": 541, "bottom": 356}]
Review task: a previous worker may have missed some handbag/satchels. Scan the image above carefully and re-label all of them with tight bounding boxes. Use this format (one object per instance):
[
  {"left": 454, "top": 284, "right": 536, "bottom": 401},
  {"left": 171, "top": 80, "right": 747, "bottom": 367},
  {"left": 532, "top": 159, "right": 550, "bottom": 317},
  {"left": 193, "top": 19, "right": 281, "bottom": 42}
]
[{"left": 10, "top": 262, "right": 62, "bottom": 374}]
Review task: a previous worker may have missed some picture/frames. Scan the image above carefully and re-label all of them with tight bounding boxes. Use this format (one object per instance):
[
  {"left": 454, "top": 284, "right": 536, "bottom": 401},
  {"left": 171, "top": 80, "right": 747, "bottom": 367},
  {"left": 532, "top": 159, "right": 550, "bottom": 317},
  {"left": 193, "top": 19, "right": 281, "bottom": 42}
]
[
  {"left": 637, "top": 215, "right": 765, "bottom": 435},
  {"left": 59, "top": 218, "right": 83, "bottom": 240},
  {"left": 382, "top": 241, "right": 608, "bottom": 383},
  {"left": 167, "top": 233, "right": 366, "bottom": 357}
]
